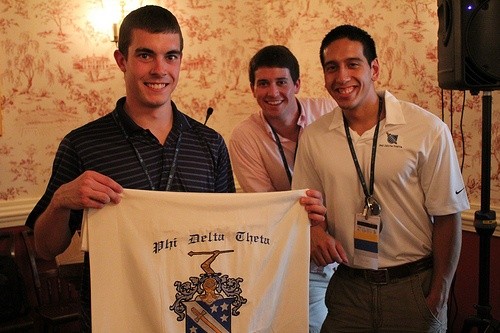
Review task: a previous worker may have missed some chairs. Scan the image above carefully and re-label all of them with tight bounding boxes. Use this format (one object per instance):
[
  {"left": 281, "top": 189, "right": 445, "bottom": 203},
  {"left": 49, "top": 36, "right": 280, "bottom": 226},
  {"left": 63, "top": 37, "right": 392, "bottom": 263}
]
[{"left": 0, "top": 227, "right": 82, "bottom": 333}]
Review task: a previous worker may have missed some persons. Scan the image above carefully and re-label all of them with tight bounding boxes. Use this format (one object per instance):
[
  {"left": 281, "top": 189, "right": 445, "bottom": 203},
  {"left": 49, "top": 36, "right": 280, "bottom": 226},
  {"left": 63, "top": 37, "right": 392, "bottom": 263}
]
[
  {"left": 228, "top": 44, "right": 341, "bottom": 332},
  {"left": 291, "top": 24, "right": 471, "bottom": 332},
  {"left": 23, "top": 4, "right": 328, "bottom": 333}
]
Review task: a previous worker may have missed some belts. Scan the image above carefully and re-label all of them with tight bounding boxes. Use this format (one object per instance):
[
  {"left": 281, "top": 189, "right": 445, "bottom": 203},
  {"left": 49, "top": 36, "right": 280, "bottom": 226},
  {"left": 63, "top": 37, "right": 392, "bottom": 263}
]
[{"left": 341, "top": 254, "right": 433, "bottom": 285}]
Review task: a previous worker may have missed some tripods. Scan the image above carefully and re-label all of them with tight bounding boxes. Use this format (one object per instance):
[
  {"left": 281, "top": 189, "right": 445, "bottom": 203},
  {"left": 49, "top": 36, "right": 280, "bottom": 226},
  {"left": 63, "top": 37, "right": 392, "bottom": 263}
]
[{"left": 458, "top": 91, "right": 500, "bottom": 333}]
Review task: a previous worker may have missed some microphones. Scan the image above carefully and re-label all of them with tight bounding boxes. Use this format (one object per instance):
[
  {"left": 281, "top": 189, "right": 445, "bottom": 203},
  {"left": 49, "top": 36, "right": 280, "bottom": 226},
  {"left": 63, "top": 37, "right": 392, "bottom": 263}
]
[{"left": 204, "top": 107, "right": 213, "bottom": 125}]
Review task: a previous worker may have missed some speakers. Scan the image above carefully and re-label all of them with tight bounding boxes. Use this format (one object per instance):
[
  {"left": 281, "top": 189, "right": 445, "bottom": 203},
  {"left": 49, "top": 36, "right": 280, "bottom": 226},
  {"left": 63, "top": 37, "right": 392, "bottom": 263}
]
[{"left": 437, "top": 0, "right": 500, "bottom": 95}]
